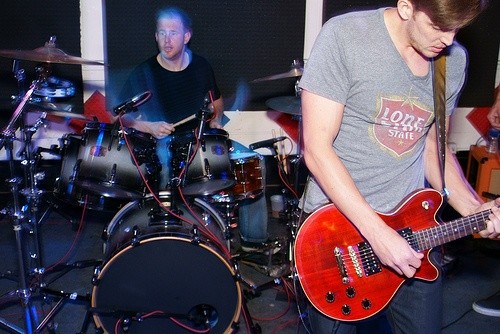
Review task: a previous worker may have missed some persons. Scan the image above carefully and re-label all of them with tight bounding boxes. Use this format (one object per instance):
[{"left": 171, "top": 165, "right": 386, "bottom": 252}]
[
  {"left": 472, "top": 47, "right": 500, "bottom": 315},
  {"left": 113, "top": 5, "right": 221, "bottom": 136},
  {"left": 298, "top": 0, "right": 500, "bottom": 334}
]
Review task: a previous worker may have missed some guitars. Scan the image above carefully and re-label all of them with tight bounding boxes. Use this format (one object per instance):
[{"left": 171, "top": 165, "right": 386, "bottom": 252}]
[{"left": 292, "top": 187, "right": 500, "bottom": 322}]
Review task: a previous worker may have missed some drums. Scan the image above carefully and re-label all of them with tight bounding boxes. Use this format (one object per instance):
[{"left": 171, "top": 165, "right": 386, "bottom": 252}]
[
  {"left": 208, "top": 151, "right": 265, "bottom": 209},
  {"left": 169, "top": 127, "right": 237, "bottom": 197},
  {"left": 52, "top": 132, "right": 107, "bottom": 212},
  {"left": 21, "top": 108, "right": 94, "bottom": 159},
  {"left": 71, "top": 121, "right": 161, "bottom": 201},
  {"left": 91, "top": 190, "right": 243, "bottom": 334}
]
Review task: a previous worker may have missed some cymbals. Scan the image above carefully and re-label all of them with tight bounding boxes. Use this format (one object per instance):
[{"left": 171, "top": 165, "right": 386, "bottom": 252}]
[
  {"left": 264, "top": 94, "right": 303, "bottom": 116},
  {"left": 0, "top": 48, "right": 106, "bottom": 66},
  {"left": 32, "top": 77, "right": 76, "bottom": 98},
  {"left": 246, "top": 68, "right": 305, "bottom": 86}
]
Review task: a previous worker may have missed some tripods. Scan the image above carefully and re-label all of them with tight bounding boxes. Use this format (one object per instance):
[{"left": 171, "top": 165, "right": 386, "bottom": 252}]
[{"left": 0, "top": 62, "right": 98, "bottom": 334}]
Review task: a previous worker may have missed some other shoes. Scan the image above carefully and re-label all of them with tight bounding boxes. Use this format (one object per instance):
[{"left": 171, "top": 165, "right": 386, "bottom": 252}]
[
  {"left": 472, "top": 289, "right": 500, "bottom": 318},
  {"left": 436, "top": 252, "right": 471, "bottom": 280}
]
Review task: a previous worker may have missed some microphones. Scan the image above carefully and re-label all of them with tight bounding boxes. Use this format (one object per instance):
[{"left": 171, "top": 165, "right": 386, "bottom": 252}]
[
  {"left": 249, "top": 137, "right": 285, "bottom": 150},
  {"left": 113, "top": 90, "right": 151, "bottom": 113}
]
